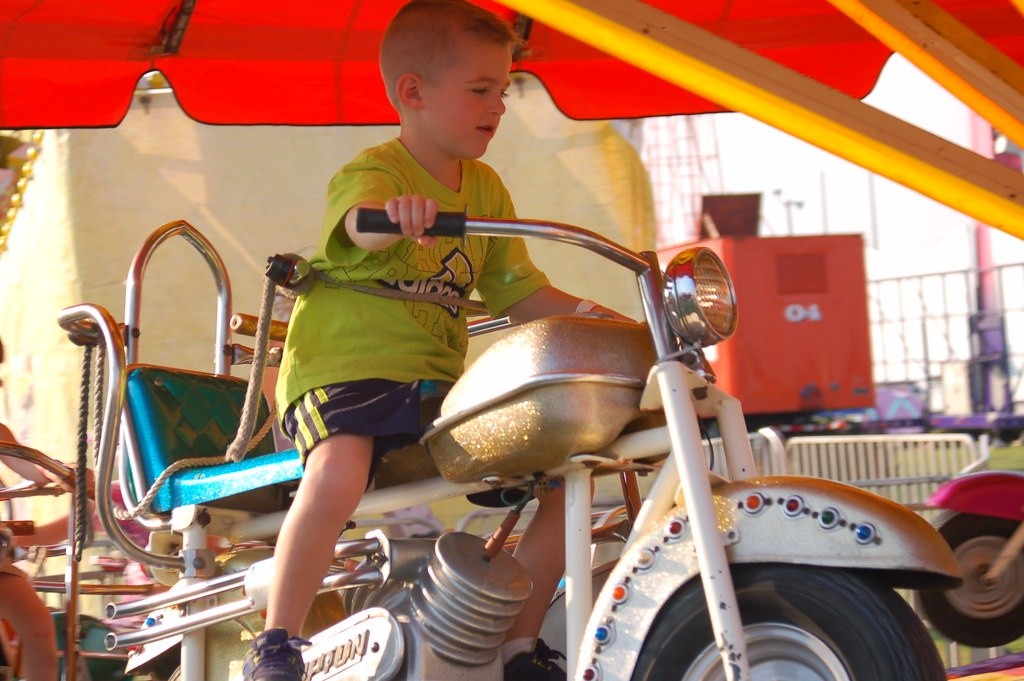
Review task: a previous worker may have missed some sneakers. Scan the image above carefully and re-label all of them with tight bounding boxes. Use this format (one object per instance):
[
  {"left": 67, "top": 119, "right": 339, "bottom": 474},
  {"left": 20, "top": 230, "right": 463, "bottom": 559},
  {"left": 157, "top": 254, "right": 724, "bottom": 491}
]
[
  {"left": 501, "top": 637, "right": 567, "bottom": 681},
  {"left": 241, "top": 627, "right": 313, "bottom": 681}
]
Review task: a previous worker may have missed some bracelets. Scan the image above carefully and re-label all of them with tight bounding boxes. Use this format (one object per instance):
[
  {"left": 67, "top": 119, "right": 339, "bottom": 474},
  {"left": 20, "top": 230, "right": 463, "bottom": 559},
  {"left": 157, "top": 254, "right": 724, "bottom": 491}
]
[{"left": 575, "top": 299, "right": 599, "bottom": 313}]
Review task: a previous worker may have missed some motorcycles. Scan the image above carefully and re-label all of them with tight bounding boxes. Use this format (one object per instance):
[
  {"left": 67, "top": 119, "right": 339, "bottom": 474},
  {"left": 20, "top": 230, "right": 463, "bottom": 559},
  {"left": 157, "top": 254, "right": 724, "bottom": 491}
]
[{"left": 0, "top": 208, "right": 1024, "bottom": 681}]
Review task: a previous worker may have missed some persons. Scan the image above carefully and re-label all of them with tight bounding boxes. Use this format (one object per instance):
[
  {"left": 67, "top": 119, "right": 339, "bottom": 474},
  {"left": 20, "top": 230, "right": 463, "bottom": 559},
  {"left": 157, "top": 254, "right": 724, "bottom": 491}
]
[
  {"left": 242, "top": 0, "right": 640, "bottom": 681},
  {"left": 0, "top": 338, "right": 93, "bottom": 681}
]
